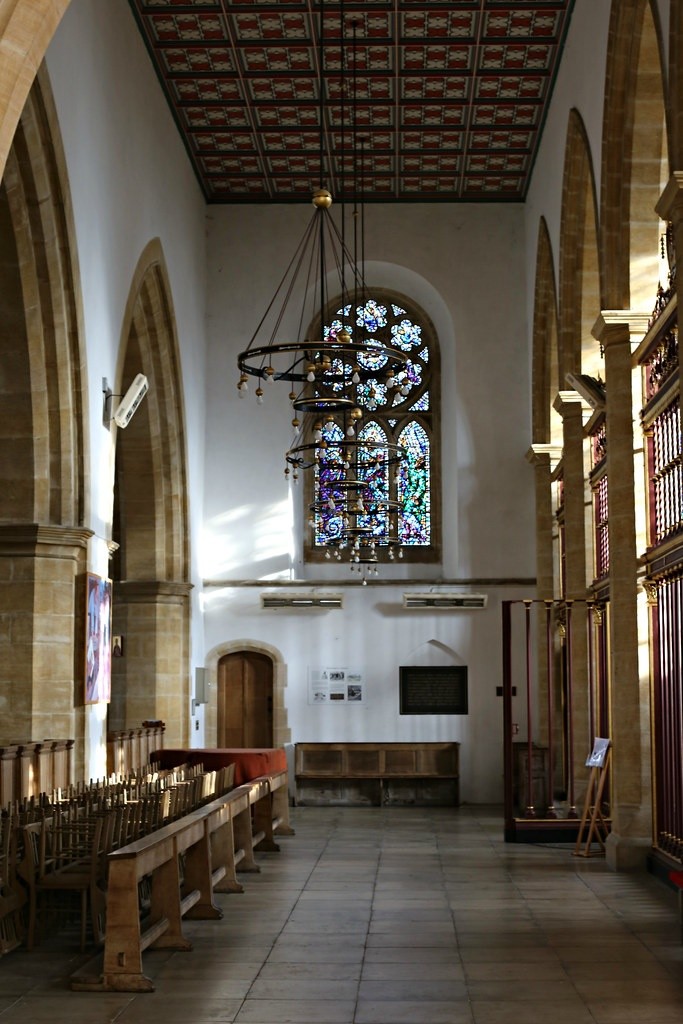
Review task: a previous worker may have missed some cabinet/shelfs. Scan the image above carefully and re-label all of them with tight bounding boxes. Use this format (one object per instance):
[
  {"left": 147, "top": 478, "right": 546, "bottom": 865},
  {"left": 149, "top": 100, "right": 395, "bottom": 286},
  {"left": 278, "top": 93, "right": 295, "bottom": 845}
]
[{"left": 512, "top": 742, "right": 555, "bottom": 818}]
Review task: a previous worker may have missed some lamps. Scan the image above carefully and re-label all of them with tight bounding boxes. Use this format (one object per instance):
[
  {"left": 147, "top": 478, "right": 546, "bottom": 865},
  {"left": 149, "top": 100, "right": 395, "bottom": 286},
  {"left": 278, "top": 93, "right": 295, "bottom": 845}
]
[
  {"left": 237, "top": 0, "right": 408, "bottom": 585},
  {"left": 103, "top": 372, "right": 149, "bottom": 432},
  {"left": 565, "top": 372, "right": 605, "bottom": 409}
]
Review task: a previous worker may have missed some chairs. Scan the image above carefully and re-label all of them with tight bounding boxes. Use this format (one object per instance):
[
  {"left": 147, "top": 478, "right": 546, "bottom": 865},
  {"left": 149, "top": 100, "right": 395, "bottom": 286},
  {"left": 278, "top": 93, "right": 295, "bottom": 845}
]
[{"left": 0, "top": 761, "right": 236, "bottom": 954}]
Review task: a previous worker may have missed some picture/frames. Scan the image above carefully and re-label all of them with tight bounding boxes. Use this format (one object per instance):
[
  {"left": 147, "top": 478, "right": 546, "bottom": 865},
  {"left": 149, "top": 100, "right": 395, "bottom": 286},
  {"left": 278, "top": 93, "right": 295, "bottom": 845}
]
[{"left": 84, "top": 571, "right": 113, "bottom": 704}]
